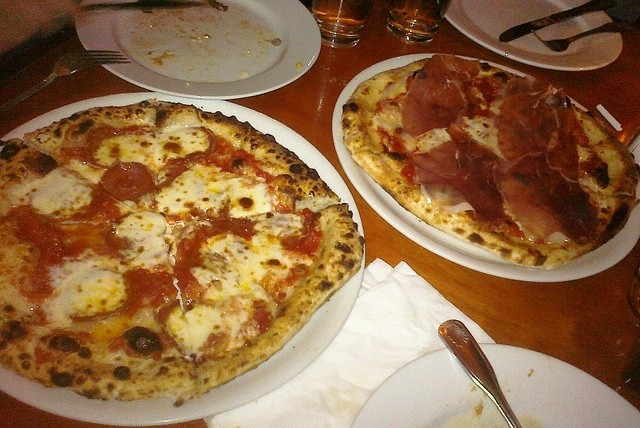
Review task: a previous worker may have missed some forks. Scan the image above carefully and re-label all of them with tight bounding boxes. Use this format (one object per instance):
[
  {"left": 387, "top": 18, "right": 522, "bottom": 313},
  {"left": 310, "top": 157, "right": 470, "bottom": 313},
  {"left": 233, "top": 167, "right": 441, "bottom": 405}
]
[
  {"left": 531, "top": 20, "right": 635, "bottom": 51},
  {"left": 1, "top": 49, "right": 130, "bottom": 111}
]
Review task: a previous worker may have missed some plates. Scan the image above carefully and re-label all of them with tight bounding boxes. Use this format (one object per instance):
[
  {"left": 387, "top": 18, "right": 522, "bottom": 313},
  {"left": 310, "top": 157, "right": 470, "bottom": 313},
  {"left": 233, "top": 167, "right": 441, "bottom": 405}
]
[
  {"left": 353, "top": 342, "right": 640, "bottom": 427},
  {"left": 75, "top": 0, "right": 322, "bottom": 101},
  {"left": 441, "top": 0, "right": 623, "bottom": 71}
]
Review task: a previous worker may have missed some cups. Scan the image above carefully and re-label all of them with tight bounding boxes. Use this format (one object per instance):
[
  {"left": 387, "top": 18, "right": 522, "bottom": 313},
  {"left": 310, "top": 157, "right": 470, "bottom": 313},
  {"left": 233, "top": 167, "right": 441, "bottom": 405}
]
[
  {"left": 386, "top": 0, "right": 450, "bottom": 45},
  {"left": 311, "top": 0, "right": 370, "bottom": 51}
]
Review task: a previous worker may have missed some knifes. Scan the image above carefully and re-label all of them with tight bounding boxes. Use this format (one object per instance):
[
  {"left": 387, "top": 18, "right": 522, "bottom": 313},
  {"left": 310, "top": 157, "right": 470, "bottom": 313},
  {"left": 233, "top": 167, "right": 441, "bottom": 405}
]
[{"left": 499, "top": 0, "right": 621, "bottom": 41}]
